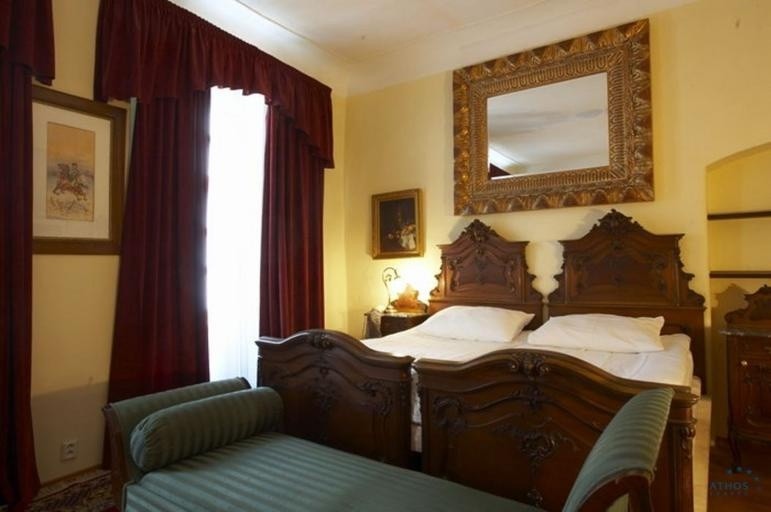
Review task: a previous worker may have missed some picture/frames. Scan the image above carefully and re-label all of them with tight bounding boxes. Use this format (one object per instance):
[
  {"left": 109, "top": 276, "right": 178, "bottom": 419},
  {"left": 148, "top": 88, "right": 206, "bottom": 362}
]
[
  {"left": 371, "top": 188, "right": 425, "bottom": 259},
  {"left": 29, "top": 83, "right": 125, "bottom": 256}
]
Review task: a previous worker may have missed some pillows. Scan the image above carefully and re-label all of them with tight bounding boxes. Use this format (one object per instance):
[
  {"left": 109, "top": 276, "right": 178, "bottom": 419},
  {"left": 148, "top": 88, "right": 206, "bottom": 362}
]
[
  {"left": 416, "top": 304, "right": 535, "bottom": 345},
  {"left": 528, "top": 314, "right": 665, "bottom": 355},
  {"left": 128, "top": 385, "right": 284, "bottom": 473}
]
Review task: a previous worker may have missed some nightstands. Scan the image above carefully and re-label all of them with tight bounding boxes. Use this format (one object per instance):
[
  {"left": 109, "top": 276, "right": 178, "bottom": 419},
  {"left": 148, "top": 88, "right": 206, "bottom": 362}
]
[
  {"left": 724, "top": 286, "right": 771, "bottom": 472},
  {"left": 380, "top": 312, "right": 431, "bottom": 338}
]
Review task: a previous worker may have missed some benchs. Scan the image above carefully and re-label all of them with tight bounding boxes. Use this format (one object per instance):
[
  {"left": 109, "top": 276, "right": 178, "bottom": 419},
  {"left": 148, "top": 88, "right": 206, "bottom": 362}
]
[{"left": 101, "top": 371, "right": 675, "bottom": 512}]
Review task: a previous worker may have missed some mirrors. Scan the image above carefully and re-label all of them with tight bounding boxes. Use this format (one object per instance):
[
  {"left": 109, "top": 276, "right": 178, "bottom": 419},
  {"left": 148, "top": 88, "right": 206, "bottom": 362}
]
[{"left": 452, "top": 18, "right": 656, "bottom": 215}]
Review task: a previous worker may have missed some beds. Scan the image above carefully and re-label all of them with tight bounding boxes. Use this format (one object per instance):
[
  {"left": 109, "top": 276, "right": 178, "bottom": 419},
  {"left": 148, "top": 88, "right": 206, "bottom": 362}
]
[{"left": 254, "top": 209, "right": 707, "bottom": 512}]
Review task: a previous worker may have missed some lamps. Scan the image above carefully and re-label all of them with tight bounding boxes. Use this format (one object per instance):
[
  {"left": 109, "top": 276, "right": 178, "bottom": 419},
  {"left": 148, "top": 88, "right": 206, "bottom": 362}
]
[{"left": 381, "top": 266, "right": 406, "bottom": 314}]
